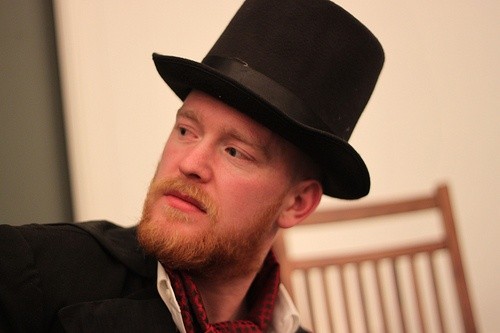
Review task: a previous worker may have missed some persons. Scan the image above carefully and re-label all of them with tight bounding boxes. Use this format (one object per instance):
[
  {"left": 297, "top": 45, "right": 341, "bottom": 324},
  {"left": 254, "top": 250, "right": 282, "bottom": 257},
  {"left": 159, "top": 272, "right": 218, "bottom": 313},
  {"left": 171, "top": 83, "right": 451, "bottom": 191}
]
[{"left": 0, "top": 0, "right": 386, "bottom": 333}]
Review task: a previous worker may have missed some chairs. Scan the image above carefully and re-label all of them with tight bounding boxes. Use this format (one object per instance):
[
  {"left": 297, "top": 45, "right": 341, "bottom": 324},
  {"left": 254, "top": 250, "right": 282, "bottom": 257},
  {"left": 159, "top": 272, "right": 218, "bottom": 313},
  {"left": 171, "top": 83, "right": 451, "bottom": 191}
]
[{"left": 271, "top": 182, "right": 477, "bottom": 332}]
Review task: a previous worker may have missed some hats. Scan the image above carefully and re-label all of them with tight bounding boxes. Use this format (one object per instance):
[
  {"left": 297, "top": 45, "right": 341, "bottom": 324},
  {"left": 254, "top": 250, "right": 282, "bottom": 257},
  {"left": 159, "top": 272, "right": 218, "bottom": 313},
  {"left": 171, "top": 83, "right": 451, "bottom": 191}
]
[{"left": 152, "top": 0, "right": 385, "bottom": 200}]
